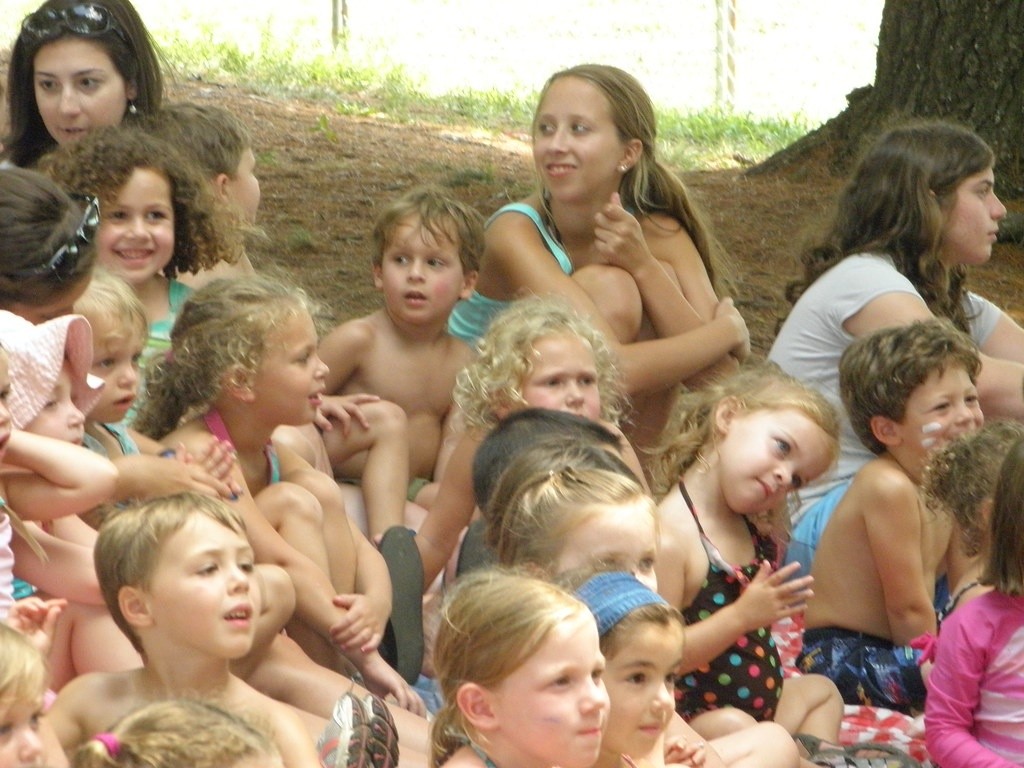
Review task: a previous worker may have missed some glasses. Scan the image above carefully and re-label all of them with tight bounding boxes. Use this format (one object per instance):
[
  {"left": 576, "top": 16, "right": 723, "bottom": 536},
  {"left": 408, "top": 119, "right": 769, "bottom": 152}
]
[
  {"left": 13, "top": 192, "right": 101, "bottom": 283},
  {"left": 22, "top": 3, "right": 127, "bottom": 46}
]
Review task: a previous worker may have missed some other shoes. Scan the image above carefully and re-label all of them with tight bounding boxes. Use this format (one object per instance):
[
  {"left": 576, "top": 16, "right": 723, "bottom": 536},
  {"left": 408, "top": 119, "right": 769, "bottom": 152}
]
[{"left": 375, "top": 525, "right": 427, "bottom": 687}]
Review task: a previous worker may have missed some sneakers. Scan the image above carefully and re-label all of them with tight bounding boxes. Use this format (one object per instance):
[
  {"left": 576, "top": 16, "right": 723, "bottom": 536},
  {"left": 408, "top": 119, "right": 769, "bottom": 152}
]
[
  {"left": 363, "top": 692, "right": 399, "bottom": 768},
  {"left": 317, "top": 692, "right": 371, "bottom": 768}
]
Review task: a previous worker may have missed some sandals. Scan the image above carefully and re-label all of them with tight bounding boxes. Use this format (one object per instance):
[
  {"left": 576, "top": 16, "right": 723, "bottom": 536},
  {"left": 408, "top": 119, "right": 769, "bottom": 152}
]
[{"left": 792, "top": 733, "right": 919, "bottom": 768}]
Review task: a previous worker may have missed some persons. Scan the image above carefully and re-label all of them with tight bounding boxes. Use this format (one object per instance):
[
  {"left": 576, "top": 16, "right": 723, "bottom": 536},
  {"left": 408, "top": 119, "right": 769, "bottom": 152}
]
[
  {"left": 315, "top": 186, "right": 478, "bottom": 499},
  {"left": 8, "top": 0, "right": 162, "bottom": 169},
  {"left": 908, "top": 434, "right": 1024, "bottom": 768},
  {"left": 765, "top": 122, "right": 1024, "bottom": 580},
  {"left": 653, "top": 356, "right": 845, "bottom": 747},
  {"left": 921, "top": 418, "right": 1024, "bottom": 628},
  {"left": 430, "top": 409, "right": 800, "bottom": 768},
  {"left": 794, "top": 324, "right": 994, "bottom": 709},
  {"left": 0, "top": 102, "right": 430, "bottom": 768},
  {"left": 448, "top": 63, "right": 751, "bottom": 456},
  {"left": 415, "top": 301, "right": 623, "bottom": 594}
]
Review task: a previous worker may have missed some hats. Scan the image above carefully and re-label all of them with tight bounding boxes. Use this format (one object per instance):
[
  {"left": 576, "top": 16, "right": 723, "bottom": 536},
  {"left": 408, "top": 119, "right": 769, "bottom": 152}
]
[{"left": 0, "top": 310, "right": 105, "bottom": 428}]
